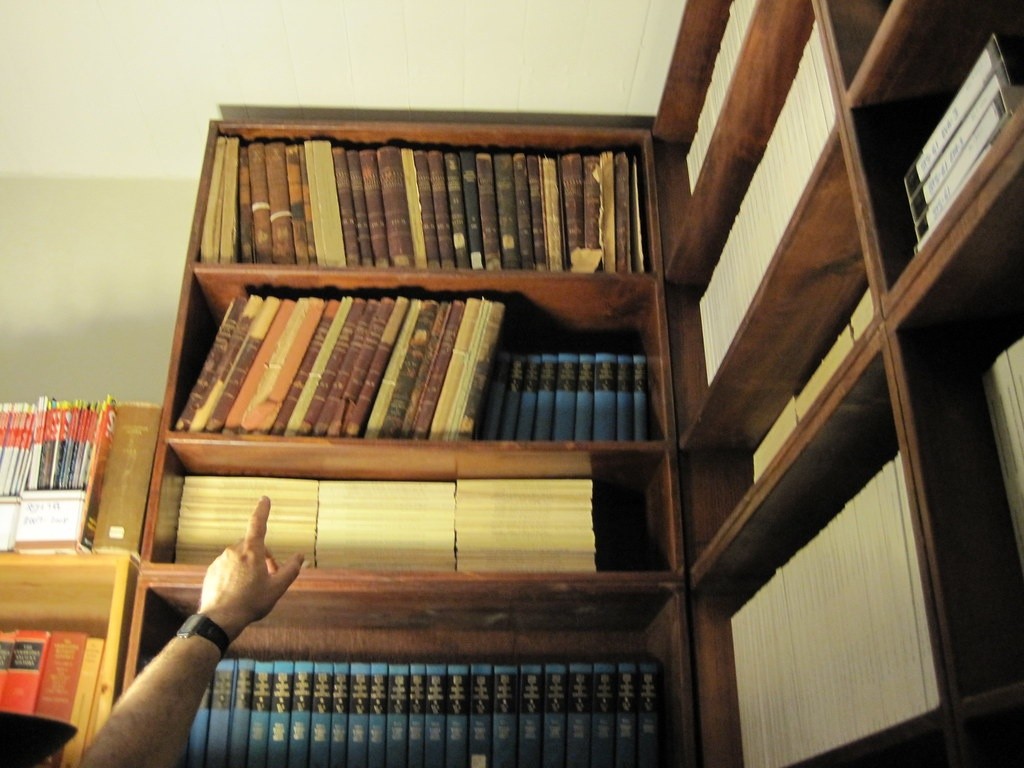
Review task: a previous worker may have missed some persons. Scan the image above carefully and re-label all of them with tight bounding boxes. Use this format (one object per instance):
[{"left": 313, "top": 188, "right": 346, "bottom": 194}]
[{"left": 79, "top": 494, "right": 303, "bottom": 768}]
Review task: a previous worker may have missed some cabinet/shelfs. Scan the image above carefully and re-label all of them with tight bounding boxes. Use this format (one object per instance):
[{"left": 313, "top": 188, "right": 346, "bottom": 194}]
[{"left": 0, "top": 0, "right": 1024, "bottom": 768}]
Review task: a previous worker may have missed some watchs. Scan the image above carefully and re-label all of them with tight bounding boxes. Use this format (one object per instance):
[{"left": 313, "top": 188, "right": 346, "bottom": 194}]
[{"left": 175, "top": 614, "right": 231, "bottom": 660}]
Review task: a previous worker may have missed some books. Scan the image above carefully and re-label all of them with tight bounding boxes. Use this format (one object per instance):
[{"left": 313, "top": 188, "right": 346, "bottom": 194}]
[
  {"left": 729, "top": 449, "right": 940, "bottom": 768},
  {"left": 683, "top": 0, "right": 759, "bottom": 197},
  {"left": 977, "top": 335, "right": 1024, "bottom": 568},
  {"left": 902, "top": 33, "right": 1024, "bottom": 255},
  {"left": 0, "top": 394, "right": 118, "bottom": 554},
  {"left": 174, "top": 475, "right": 597, "bottom": 573},
  {"left": 0, "top": 628, "right": 106, "bottom": 768},
  {"left": 175, "top": 293, "right": 647, "bottom": 441},
  {"left": 198, "top": 136, "right": 647, "bottom": 275},
  {"left": 699, "top": 19, "right": 835, "bottom": 386},
  {"left": 183, "top": 660, "right": 658, "bottom": 767}
]
